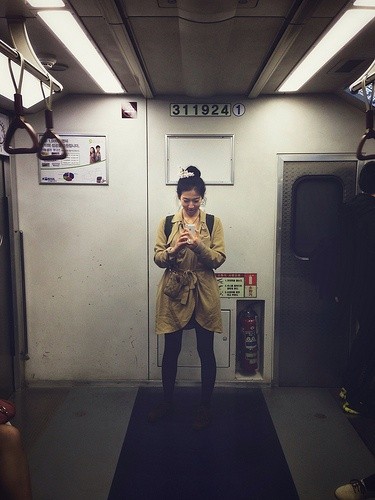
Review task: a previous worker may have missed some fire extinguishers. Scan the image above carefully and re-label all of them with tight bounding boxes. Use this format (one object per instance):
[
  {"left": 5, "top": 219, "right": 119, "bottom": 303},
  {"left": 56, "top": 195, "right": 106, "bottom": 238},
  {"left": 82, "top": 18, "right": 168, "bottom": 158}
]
[{"left": 237, "top": 301, "right": 259, "bottom": 370}]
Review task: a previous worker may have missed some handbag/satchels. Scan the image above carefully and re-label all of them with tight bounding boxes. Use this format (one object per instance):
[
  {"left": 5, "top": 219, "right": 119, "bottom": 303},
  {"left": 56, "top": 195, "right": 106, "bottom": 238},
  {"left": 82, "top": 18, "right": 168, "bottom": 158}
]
[{"left": 162, "top": 272, "right": 188, "bottom": 300}]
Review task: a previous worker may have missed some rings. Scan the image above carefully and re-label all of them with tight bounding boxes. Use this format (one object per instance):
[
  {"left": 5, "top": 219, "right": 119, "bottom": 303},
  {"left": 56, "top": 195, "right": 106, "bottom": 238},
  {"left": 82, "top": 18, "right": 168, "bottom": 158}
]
[{"left": 189, "top": 239, "right": 190, "bottom": 242}]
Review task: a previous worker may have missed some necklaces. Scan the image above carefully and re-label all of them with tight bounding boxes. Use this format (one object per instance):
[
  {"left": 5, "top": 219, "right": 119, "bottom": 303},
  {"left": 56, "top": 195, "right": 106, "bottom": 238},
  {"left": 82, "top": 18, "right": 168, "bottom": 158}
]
[{"left": 184, "top": 215, "right": 199, "bottom": 225}]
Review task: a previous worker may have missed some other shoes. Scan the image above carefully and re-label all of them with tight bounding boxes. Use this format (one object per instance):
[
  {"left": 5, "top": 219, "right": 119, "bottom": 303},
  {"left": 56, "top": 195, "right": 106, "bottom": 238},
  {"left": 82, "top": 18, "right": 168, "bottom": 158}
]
[
  {"left": 335, "top": 470, "right": 375, "bottom": 500},
  {"left": 338, "top": 387, "right": 348, "bottom": 400},
  {"left": 152, "top": 400, "right": 176, "bottom": 411},
  {"left": 194, "top": 408, "right": 209, "bottom": 423},
  {"left": 343, "top": 400, "right": 361, "bottom": 414}
]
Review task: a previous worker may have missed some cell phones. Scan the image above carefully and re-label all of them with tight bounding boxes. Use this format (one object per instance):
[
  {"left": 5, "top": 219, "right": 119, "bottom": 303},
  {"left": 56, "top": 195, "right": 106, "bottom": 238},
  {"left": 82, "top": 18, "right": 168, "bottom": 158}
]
[{"left": 185, "top": 224, "right": 195, "bottom": 239}]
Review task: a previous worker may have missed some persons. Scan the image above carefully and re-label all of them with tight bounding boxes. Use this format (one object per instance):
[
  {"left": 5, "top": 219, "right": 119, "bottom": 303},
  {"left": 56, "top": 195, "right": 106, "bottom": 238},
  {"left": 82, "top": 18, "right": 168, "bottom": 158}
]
[
  {"left": 337, "top": 161, "right": 375, "bottom": 418},
  {"left": 90, "top": 145, "right": 101, "bottom": 164},
  {"left": 334, "top": 473, "right": 375, "bottom": 500},
  {"left": 145, "top": 166, "right": 226, "bottom": 429}
]
